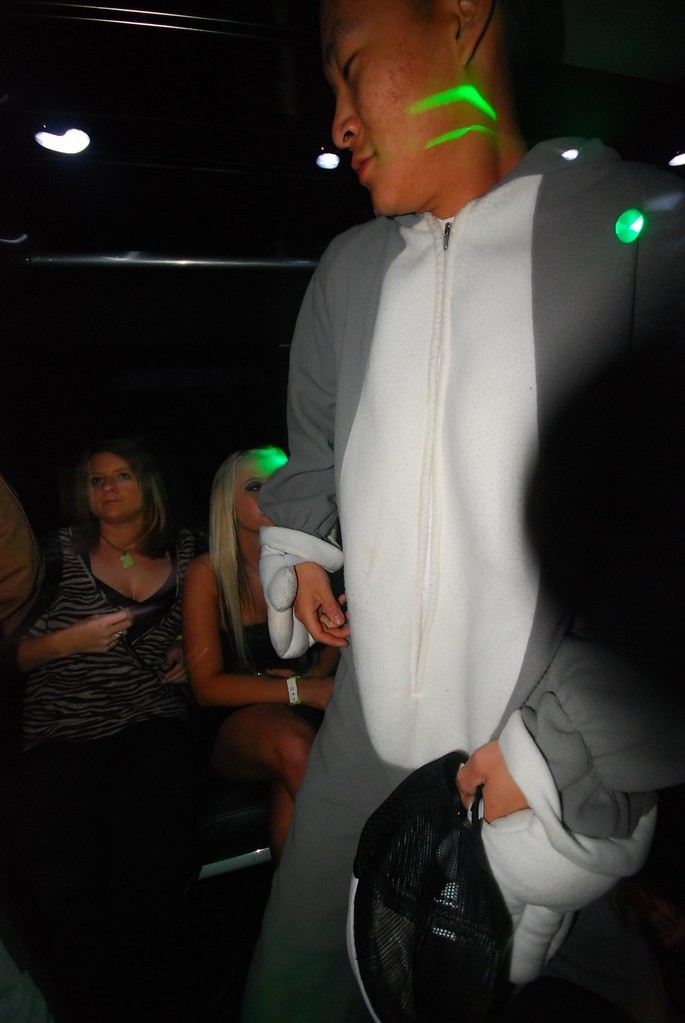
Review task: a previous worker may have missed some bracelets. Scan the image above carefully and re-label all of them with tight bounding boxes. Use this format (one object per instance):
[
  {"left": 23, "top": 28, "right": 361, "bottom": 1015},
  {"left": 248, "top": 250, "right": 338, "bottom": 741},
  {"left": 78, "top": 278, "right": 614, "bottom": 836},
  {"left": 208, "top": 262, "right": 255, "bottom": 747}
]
[{"left": 285, "top": 675, "right": 302, "bottom": 705}]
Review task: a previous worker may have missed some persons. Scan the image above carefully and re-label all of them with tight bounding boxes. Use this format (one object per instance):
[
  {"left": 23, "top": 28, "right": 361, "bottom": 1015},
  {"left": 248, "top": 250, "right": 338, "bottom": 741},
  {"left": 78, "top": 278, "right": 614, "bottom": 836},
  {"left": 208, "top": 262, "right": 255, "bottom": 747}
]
[
  {"left": 0, "top": 440, "right": 215, "bottom": 1023},
  {"left": 183, "top": 443, "right": 349, "bottom": 872},
  {"left": 244, "top": 1, "right": 685, "bottom": 1023}
]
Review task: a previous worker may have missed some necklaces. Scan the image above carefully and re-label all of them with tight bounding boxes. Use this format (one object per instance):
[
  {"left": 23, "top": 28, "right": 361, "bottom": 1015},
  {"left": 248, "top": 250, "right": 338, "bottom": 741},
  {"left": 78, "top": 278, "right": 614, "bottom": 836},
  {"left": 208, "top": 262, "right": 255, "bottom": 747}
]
[{"left": 99, "top": 529, "right": 146, "bottom": 569}]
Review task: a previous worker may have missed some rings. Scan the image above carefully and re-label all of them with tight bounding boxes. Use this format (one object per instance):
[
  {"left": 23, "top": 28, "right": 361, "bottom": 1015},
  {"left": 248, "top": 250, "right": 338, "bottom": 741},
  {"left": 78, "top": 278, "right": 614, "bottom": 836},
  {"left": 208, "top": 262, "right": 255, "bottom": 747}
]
[
  {"left": 116, "top": 639, "right": 121, "bottom": 645},
  {"left": 114, "top": 632, "right": 124, "bottom": 638}
]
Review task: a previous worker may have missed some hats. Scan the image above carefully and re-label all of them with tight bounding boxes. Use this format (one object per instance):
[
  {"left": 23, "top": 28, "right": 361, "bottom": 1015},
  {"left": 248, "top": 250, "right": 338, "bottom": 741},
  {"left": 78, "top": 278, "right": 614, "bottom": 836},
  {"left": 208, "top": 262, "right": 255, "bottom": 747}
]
[{"left": 346, "top": 750, "right": 512, "bottom": 1023}]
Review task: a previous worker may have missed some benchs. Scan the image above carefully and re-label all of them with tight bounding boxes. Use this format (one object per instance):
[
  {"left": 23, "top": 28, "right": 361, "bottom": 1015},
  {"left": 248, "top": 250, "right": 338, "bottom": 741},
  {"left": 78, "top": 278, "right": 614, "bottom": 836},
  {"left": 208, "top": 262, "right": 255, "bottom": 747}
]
[{"left": 195, "top": 779, "right": 270, "bottom": 880}]
